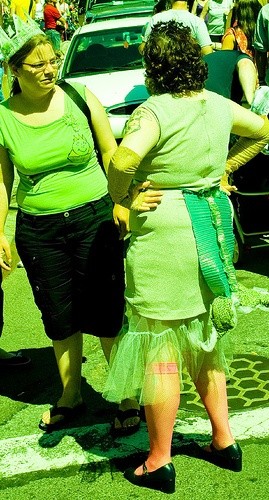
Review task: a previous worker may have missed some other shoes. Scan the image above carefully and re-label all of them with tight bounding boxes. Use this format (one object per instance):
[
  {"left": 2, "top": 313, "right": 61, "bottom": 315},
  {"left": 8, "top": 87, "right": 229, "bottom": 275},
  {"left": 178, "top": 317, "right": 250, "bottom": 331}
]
[{"left": 0, "top": 351, "right": 31, "bottom": 365}]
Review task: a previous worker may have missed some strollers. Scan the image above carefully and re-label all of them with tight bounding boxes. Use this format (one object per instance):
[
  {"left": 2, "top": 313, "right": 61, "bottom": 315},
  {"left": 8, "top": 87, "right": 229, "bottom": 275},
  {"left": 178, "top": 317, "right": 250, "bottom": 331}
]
[{"left": 229, "top": 141, "right": 269, "bottom": 265}]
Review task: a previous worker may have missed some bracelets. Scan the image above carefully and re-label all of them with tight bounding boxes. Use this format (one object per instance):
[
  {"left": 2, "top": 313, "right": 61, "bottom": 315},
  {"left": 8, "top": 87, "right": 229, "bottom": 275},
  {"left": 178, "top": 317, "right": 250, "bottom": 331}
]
[{"left": 215, "top": 42, "right": 216, "bottom": 49}]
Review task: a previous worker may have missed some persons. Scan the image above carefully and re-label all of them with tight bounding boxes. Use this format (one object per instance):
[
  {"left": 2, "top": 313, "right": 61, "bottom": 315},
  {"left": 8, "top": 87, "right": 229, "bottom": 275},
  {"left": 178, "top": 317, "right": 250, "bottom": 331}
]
[
  {"left": 102, "top": 20, "right": 269, "bottom": 492},
  {"left": 0, "top": 7, "right": 141, "bottom": 435},
  {"left": 0, "top": 0, "right": 269, "bottom": 366}
]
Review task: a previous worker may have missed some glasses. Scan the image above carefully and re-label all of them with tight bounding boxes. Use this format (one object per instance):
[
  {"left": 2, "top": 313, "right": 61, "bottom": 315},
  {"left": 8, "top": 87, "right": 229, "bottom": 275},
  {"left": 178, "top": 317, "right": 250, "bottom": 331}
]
[{"left": 22, "top": 58, "right": 62, "bottom": 70}]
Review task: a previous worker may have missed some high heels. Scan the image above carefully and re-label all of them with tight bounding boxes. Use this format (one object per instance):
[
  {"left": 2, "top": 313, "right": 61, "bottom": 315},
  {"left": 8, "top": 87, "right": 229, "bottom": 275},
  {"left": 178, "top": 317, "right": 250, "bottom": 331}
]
[
  {"left": 124, "top": 461, "right": 176, "bottom": 495},
  {"left": 197, "top": 442, "right": 242, "bottom": 472}
]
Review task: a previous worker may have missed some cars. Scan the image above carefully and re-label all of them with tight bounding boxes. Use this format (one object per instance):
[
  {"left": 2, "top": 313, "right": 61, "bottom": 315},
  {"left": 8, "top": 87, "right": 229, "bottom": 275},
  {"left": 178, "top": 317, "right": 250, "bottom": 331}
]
[
  {"left": 58, "top": 17, "right": 152, "bottom": 146},
  {"left": 84, "top": 0, "right": 159, "bottom": 25}
]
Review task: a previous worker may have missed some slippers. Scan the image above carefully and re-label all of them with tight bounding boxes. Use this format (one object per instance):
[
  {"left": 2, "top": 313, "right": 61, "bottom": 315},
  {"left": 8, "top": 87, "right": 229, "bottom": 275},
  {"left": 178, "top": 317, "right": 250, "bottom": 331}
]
[
  {"left": 39, "top": 395, "right": 83, "bottom": 430},
  {"left": 109, "top": 407, "right": 141, "bottom": 437}
]
